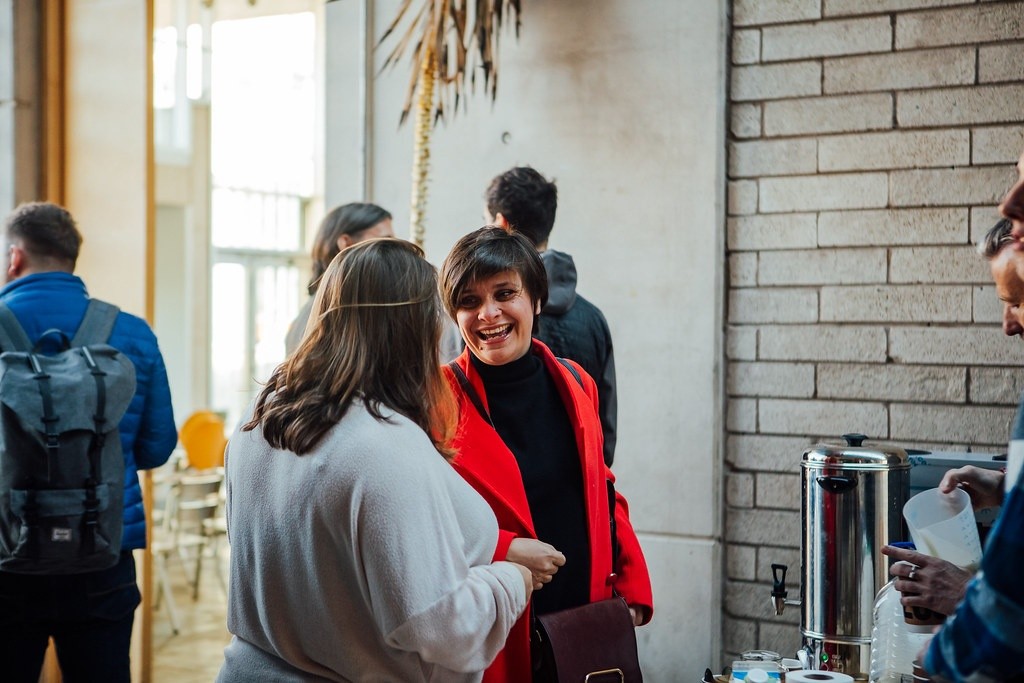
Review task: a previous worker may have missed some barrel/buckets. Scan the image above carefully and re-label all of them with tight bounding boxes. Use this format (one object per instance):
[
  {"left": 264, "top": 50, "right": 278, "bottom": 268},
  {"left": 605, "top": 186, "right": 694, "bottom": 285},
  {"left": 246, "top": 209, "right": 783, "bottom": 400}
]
[{"left": 770, "top": 432, "right": 914, "bottom": 683}]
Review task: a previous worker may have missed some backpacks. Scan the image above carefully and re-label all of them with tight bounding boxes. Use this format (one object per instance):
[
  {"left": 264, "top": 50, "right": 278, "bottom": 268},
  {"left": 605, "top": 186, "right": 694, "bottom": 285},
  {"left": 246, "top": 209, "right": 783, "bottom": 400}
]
[{"left": 0, "top": 298, "right": 136, "bottom": 574}]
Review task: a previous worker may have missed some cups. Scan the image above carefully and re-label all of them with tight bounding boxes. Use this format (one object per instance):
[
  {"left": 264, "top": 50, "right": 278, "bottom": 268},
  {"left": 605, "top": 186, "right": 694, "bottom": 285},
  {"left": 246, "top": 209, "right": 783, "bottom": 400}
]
[
  {"left": 740, "top": 649, "right": 782, "bottom": 665},
  {"left": 895, "top": 561, "right": 947, "bottom": 633},
  {"left": 912, "top": 660, "right": 931, "bottom": 683}
]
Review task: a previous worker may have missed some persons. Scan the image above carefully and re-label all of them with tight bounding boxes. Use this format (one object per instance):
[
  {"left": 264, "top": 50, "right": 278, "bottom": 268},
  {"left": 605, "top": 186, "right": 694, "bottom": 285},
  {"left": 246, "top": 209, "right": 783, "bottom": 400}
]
[
  {"left": 0, "top": 203, "right": 178, "bottom": 683},
  {"left": 427, "top": 167, "right": 657, "bottom": 683},
  {"left": 216, "top": 202, "right": 534, "bottom": 683},
  {"left": 881, "top": 150, "right": 1024, "bottom": 683}
]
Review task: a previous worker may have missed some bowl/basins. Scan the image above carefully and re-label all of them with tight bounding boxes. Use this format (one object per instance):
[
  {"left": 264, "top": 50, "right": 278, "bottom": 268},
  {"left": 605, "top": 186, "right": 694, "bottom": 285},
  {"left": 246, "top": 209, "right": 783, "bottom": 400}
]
[{"left": 779, "top": 657, "right": 803, "bottom": 679}]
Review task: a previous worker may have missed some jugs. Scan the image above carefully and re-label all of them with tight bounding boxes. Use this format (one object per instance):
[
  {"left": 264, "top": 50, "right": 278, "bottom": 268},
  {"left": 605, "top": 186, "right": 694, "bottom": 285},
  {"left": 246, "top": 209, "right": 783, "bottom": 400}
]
[{"left": 903, "top": 486, "right": 984, "bottom": 576}]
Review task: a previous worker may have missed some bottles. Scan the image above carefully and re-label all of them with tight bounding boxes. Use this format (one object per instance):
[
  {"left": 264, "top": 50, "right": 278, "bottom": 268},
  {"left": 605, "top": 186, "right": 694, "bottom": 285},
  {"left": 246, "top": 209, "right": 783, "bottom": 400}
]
[{"left": 869, "top": 576, "right": 935, "bottom": 683}]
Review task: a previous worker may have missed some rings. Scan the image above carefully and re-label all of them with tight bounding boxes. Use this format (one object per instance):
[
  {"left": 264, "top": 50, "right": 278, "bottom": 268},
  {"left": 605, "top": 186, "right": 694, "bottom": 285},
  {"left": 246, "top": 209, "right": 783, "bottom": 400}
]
[{"left": 909, "top": 566, "right": 916, "bottom": 579}]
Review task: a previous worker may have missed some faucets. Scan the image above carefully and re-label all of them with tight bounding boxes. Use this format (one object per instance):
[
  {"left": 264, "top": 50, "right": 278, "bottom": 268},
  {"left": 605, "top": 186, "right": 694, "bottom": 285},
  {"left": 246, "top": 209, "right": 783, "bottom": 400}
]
[{"left": 768, "top": 561, "right": 789, "bottom": 617}]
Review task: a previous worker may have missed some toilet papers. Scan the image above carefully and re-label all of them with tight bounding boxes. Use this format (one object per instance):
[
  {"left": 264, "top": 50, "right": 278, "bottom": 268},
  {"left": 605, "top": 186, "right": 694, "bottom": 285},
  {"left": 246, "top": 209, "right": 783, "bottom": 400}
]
[{"left": 784, "top": 670, "right": 855, "bottom": 683}]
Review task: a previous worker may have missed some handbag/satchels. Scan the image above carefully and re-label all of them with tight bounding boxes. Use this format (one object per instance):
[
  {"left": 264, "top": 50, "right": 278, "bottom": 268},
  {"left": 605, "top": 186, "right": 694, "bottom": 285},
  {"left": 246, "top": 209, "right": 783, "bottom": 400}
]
[{"left": 532, "top": 596, "right": 643, "bottom": 683}]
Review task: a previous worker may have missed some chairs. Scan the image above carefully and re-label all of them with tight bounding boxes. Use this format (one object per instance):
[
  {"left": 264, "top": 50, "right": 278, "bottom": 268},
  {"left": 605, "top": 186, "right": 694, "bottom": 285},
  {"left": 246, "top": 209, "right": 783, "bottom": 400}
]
[{"left": 131, "top": 468, "right": 230, "bottom": 634}]
